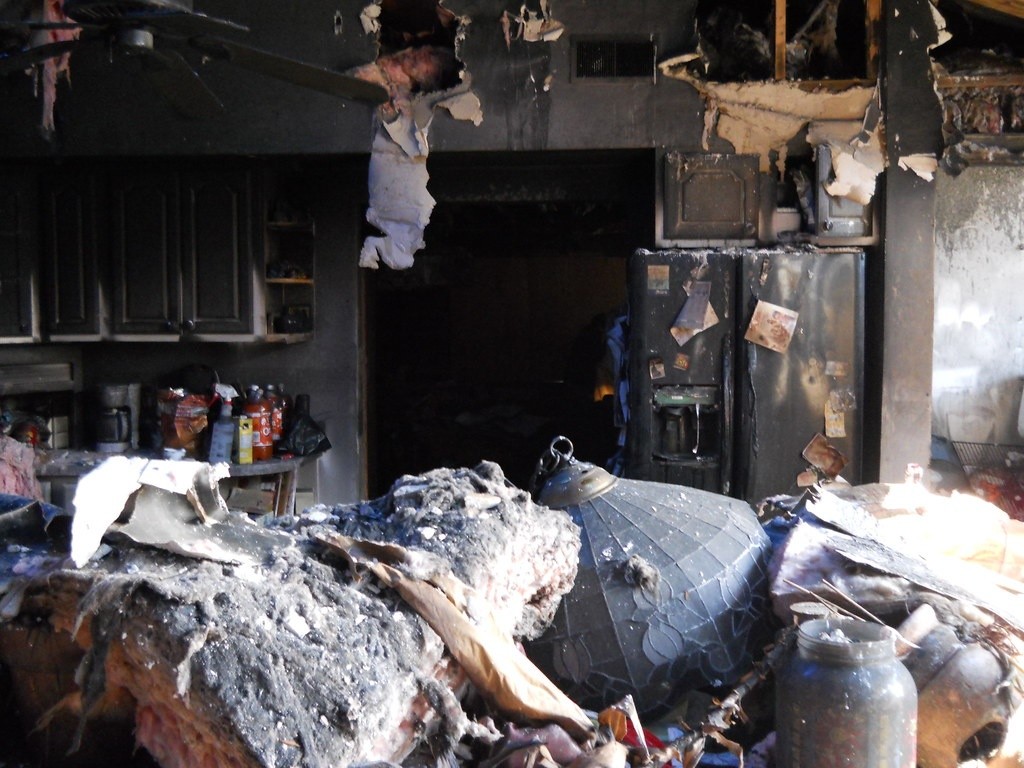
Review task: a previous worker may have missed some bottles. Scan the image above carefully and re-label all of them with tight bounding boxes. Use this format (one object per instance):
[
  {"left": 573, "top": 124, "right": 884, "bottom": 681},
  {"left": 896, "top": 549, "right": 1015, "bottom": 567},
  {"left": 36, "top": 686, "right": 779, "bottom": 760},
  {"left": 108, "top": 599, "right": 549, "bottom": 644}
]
[
  {"left": 244, "top": 383, "right": 291, "bottom": 461},
  {"left": 775, "top": 602, "right": 919, "bottom": 768}
]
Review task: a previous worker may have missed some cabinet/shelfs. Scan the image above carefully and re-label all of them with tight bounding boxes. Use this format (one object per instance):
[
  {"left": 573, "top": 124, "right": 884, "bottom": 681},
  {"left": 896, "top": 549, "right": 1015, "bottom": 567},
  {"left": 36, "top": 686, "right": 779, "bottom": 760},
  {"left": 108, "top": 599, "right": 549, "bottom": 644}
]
[
  {"left": 104, "top": 158, "right": 316, "bottom": 345},
  {"left": 0, "top": 160, "right": 105, "bottom": 345}
]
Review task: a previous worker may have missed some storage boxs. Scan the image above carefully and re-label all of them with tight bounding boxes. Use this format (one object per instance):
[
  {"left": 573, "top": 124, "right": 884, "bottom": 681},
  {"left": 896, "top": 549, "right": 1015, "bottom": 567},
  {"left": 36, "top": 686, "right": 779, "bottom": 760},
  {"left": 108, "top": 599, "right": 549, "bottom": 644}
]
[{"left": 232, "top": 415, "right": 252, "bottom": 466}]
